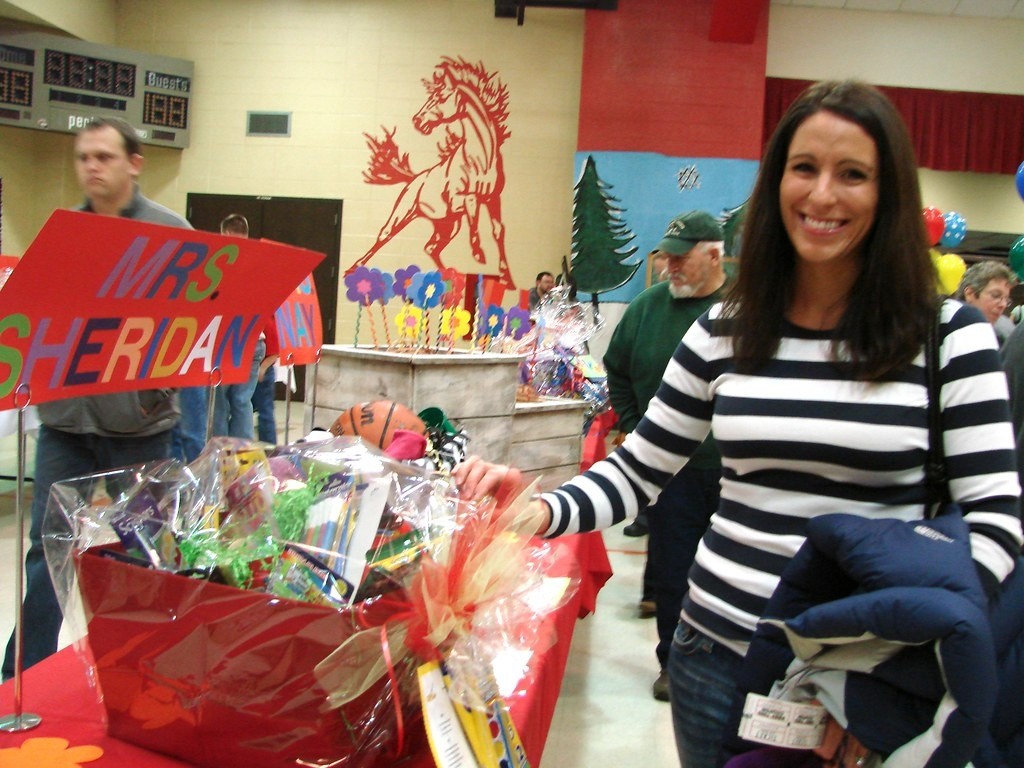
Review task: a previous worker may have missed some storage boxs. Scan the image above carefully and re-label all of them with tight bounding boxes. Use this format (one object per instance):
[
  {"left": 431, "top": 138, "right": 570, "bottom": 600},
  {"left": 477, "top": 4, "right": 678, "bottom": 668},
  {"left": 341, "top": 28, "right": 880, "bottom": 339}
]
[{"left": 66, "top": 489, "right": 525, "bottom": 768}]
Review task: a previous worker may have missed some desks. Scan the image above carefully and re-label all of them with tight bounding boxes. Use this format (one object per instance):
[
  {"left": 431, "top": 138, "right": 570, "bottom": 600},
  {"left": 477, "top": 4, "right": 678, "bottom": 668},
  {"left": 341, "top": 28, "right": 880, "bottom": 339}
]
[{"left": 0, "top": 531, "right": 613, "bottom": 768}]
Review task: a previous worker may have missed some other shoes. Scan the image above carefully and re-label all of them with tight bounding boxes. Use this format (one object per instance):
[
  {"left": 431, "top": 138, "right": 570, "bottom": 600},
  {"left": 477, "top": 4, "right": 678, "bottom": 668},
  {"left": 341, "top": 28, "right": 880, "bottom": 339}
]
[
  {"left": 653, "top": 671, "right": 669, "bottom": 703},
  {"left": 637, "top": 596, "right": 656, "bottom": 616},
  {"left": 624, "top": 520, "right": 647, "bottom": 538}
]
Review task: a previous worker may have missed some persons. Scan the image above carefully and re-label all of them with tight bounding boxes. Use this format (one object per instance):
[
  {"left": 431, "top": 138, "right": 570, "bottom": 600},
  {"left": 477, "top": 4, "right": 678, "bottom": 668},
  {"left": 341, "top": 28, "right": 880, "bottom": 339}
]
[
  {"left": 212, "top": 213, "right": 280, "bottom": 442},
  {"left": 528, "top": 272, "right": 556, "bottom": 306},
  {"left": 1, "top": 116, "right": 198, "bottom": 682},
  {"left": 605, "top": 210, "right": 754, "bottom": 702},
  {"left": 937, "top": 261, "right": 1024, "bottom": 768},
  {"left": 450, "top": 79, "right": 1024, "bottom": 768},
  {"left": 649, "top": 248, "right": 667, "bottom": 280}
]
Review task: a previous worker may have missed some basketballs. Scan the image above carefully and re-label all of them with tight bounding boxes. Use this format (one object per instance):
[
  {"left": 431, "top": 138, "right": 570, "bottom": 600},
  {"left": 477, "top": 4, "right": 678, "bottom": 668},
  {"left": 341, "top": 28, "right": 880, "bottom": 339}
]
[{"left": 329, "top": 400, "right": 429, "bottom": 452}]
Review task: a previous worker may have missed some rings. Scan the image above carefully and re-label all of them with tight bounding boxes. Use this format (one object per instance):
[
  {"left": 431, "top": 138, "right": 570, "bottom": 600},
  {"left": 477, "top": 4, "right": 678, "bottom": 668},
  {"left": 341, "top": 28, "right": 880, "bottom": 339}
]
[{"left": 855, "top": 755, "right": 864, "bottom": 766}]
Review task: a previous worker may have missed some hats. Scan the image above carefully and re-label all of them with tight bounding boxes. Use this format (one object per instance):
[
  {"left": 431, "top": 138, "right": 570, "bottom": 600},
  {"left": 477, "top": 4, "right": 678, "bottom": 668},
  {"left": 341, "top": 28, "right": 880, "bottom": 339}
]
[{"left": 649, "top": 209, "right": 725, "bottom": 257}]
